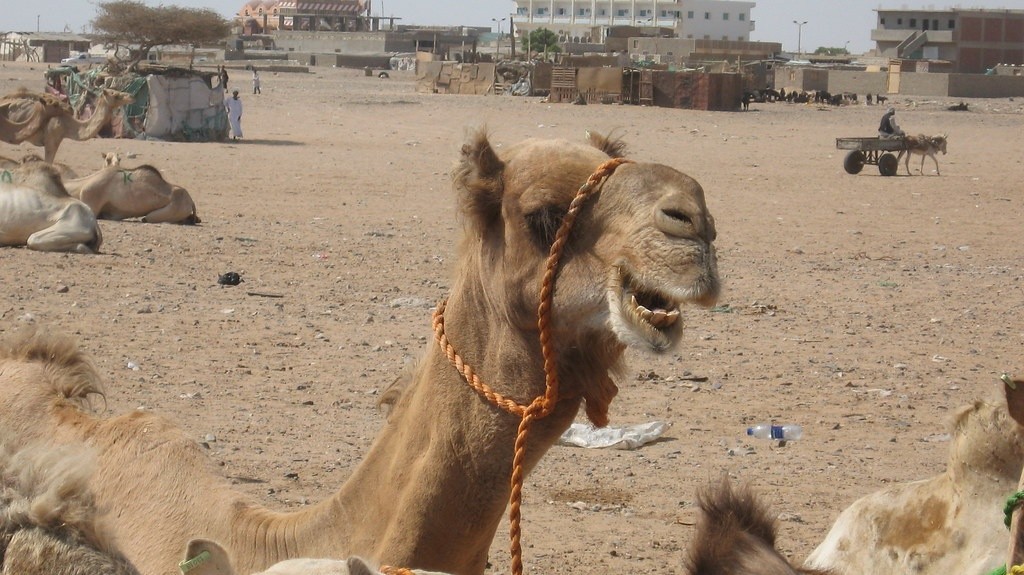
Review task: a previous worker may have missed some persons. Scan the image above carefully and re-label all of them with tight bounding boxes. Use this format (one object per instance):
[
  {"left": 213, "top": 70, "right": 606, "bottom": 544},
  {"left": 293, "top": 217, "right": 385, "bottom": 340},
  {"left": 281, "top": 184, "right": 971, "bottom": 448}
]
[
  {"left": 743, "top": 88, "right": 749, "bottom": 111},
  {"left": 252, "top": 68, "right": 261, "bottom": 94},
  {"left": 225, "top": 87, "right": 243, "bottom": 139},
  {"left": 220, "top": 65, "right": 229, "bottom": 92},
  {"left": 877, "top": 107, "right": 905, "bottom": 137}
]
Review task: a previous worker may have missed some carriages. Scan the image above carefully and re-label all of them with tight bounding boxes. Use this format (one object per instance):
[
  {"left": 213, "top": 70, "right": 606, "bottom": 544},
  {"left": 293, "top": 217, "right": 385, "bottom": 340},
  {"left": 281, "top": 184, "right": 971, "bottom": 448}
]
[{"left": 836, "top": 133, "right": 948, "bottom": 177}]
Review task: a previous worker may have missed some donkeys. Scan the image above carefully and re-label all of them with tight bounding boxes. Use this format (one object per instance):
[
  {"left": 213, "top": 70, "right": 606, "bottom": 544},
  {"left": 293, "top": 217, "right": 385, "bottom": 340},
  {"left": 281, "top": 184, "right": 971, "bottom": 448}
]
[{"left": 897, "top": 131, "right": 949, "bottom": 177}]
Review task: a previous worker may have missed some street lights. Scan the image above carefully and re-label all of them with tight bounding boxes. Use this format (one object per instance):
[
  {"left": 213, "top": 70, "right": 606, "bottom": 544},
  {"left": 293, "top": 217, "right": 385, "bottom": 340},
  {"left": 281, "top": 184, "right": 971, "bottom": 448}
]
[
  {"left": 793, "top": 20, "right": 807, "bottom": 59},
  {"left": 37, "top": 15, "right": 40, "bottom": 32},
  {"left": 845, "top": 41, "right": 850, "bottom": 49},
  {"left": 492, "top": 18, "right": 506, "bottom": 52}
]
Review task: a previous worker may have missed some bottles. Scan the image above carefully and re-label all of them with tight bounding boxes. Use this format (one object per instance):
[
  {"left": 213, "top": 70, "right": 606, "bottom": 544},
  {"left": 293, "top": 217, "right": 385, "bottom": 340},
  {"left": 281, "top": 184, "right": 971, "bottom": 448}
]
[{"left": 746, "top": 424, "right": 801, "bottom": 440}]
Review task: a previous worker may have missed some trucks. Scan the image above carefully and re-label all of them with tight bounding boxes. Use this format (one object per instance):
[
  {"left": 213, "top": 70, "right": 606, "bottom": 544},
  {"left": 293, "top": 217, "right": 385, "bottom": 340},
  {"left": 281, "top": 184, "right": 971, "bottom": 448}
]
[{"left": 61, "top": 53, "right": 109, "bottom": 64}]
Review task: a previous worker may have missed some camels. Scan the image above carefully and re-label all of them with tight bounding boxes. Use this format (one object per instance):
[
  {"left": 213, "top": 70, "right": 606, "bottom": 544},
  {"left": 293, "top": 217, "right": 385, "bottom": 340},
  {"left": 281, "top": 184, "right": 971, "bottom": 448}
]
[
  {"left": 0, "top": 87, "right": 202, "bottom": 255},
  {"left": 2, "top": 124, "right": 1024, "bottom": 575}
]
